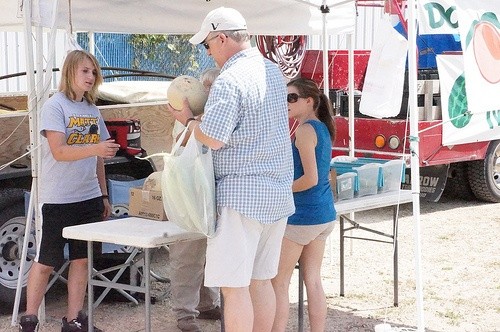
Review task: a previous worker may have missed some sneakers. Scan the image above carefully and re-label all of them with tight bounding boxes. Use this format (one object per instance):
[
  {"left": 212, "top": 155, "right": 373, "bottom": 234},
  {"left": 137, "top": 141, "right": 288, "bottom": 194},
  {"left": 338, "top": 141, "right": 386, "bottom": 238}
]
[
  {"left": 19, "top": 315, "right": 40, "bottom": 332},
  {"left": 196, "top": 305, "right": 221, "bottom": 321},
  {"left": 61, "top": 314, "right": 102, "bottom": 332},
  {"left": 177, "top": 315, "right": 200, "bottom": 331}
]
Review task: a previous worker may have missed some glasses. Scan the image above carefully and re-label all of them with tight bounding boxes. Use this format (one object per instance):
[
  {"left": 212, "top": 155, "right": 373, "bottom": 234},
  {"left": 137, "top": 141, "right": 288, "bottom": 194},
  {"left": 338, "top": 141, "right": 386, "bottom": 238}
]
[
  {"left": 287, "top": 93, "right": 307, "bottom": 103},
  {"left": 202, "top": 35, "right": 228, "bottom": 49}
]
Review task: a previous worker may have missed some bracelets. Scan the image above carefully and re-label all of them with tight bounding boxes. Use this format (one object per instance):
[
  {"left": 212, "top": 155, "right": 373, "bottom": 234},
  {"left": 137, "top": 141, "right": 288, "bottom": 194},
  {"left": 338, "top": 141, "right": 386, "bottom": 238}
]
[{"left": 185, "top": 118, "right": 195, "bottom": 127}]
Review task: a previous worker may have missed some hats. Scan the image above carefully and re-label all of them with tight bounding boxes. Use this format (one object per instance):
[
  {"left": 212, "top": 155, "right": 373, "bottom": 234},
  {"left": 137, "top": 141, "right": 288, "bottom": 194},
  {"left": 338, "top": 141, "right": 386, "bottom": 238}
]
[{"left": 189, "top": 8, "right": 247, "bottom": 45}]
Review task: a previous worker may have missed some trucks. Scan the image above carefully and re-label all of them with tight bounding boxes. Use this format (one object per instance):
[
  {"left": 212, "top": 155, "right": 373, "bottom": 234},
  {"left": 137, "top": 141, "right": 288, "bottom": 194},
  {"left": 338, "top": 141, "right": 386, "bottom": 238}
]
[
  {"left": 260, "top": 0, "right": 500, "bottom": 209},
  {"left": 3, "top": 0, "right": 336, "bottom": 310}
]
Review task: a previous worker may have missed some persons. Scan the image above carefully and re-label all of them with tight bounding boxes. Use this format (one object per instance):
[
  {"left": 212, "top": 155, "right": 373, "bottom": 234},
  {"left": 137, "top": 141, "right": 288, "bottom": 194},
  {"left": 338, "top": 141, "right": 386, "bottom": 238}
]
[
  {"left": 271, "top": 78, "right": 338, "bottom": 331},
  {"left": 19, "top": 48, "right": 121, "bottom": 332},
  {"left": 168, "top": 8, "right": 296, "bottom": 332},
  {"left": 161, "top": 68, "right": 225, "bottom": 332}
]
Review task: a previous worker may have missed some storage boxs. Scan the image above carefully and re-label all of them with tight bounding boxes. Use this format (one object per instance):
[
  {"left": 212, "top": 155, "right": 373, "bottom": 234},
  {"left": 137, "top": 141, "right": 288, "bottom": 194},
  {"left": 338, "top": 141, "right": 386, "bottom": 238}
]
[
  {"left": 127, "top": 184, "right": 169, "bottom": 221},
  {"left": 329, "top": 155, "right": 406, "bottom": 201},
  {"left": 93, "top": 252, "right": 143, "bottom": 302}
]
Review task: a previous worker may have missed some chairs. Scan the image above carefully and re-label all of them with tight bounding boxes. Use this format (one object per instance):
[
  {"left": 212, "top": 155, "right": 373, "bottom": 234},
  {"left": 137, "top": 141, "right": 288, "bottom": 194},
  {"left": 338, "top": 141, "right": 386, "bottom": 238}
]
[{"left": 22, "top": 178, "right": 174, "bottom": 317}]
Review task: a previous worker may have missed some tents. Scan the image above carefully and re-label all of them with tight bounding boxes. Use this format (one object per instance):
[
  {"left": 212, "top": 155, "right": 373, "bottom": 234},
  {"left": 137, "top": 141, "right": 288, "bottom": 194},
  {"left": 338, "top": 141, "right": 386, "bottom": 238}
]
[{"left": 0, "top": 0, "right": 426, "bottom": 332}]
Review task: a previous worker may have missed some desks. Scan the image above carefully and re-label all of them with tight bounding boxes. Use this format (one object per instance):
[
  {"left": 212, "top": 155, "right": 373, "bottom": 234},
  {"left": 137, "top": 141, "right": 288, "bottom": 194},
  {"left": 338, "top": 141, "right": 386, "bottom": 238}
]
[{"left": 60, "top": 188, "right": 414, "bottom": 332}]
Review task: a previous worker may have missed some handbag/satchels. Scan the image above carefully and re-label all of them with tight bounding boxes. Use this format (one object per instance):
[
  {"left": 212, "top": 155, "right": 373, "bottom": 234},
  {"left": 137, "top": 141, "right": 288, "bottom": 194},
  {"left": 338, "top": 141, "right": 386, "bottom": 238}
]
[{"left": 161, "top": 121, "right": 216, "bottom": 238}]
